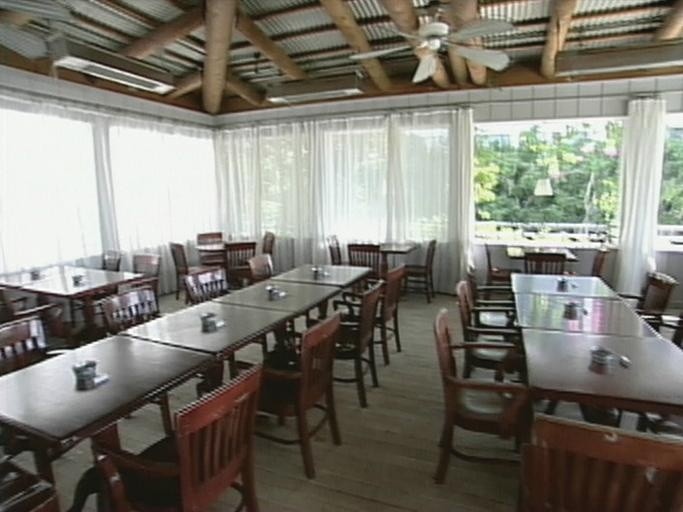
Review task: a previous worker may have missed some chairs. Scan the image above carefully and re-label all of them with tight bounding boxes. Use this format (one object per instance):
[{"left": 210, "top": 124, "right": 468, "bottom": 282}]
[
  {"left": 591, "top": 249, "right": 609, "bottom": 277},
  {"left": 518, "top": 415, "right": 683, "bottom": 511},
  {"left": 0, "top": 455, "right": 60, "bottom": 511},
  {"left": 333, "top": 266, "right": 401, "bottom": 409},
  {"left": 102, "top": 250, "right": 160, "bottom": 337},
  {"left": 0, "top": 289, "right": 71, "bottom": 378},
  {"left": 434, "top": 280, "right": 536, "bottom": 486},
  {"left": 402, "top": 240, "right": 436, "bottom": 302},
  {"left": 257, "top": 313, "right": 342, "bottom": 481},
  {"left": 91, "top": 365, "right": 257, "bottom": 512},
  {"left": 171, "top": 230, "right": 274, "bottom": 305},
  {"left": 327, "top": 235, "right": 383, "bottom": 274},
  {"left": 617, "top": 272, "right": 683, "bottom": 347},
  {"left": 485, "top": 244, "right": 520, "bottom": 303}
]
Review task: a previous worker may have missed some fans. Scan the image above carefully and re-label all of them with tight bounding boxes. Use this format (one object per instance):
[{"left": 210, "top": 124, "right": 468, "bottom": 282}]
[{"left": 350, "top": 0, "right": 516, "bottom": 90}]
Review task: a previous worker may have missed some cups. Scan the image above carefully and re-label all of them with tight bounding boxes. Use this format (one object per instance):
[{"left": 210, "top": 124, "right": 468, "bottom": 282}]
[
  {"left": 312, "top": 266, "right": 323, "bottom": 279},
  {"left": 265, "top": 284, "right": 281, "bottom": 295},
  {"left": 200, "top": 312, "right": 216, "bottom": 327},
  {"left": 558, "top": 277, "right": 570, "bottom": 287},
  {"left": 73, "top": 362, "right": 98, "bottom": 381},
  {"left": 28, "top": 268, "right": 86, "bottom": 283},
  {"left": 589, "top": 347, "right": 616, "bottom": 365},
  {"left": 565, "top": 299, "right": 583, "bottom": 316}
]
